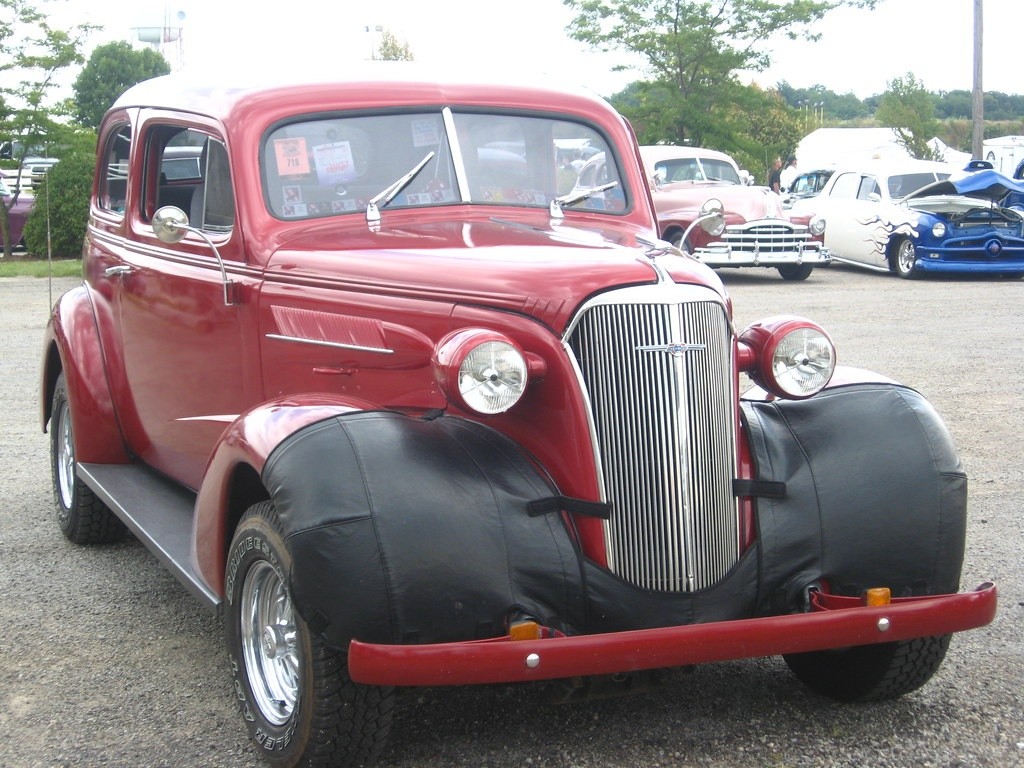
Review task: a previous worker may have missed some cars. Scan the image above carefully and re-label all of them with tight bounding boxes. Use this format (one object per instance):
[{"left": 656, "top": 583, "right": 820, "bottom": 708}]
[
  {"left": 787, "top": 158, "right": 1024, "bottom": 281},
  {"left": 561, "top": 143, "right": 835, "bottom": 286},
  {"left": 30, "top": 75, "right": 1001, "bottom": 768},
  {"left": 777, "top": 125, "right": 1024, "bottom": 204},
  {"left": 0, "top": 143, "right": 598, "bottom": 255}
]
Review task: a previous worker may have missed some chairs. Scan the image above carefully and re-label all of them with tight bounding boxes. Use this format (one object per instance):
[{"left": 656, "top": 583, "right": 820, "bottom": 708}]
[
  {"left": 108, "top": 172, "right": 195, "bottom": 221},
  {"left": 191, "top": 138, "right": 235, "bottom": 230}
]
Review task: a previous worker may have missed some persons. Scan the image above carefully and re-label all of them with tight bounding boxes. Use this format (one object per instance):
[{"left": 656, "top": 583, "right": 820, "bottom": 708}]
[
  {"left": 768, "top": 155, "right": 801, "bottom": 196},
  {"left": 803, "top": 176, "right": 814, "bottom": 192}
]
